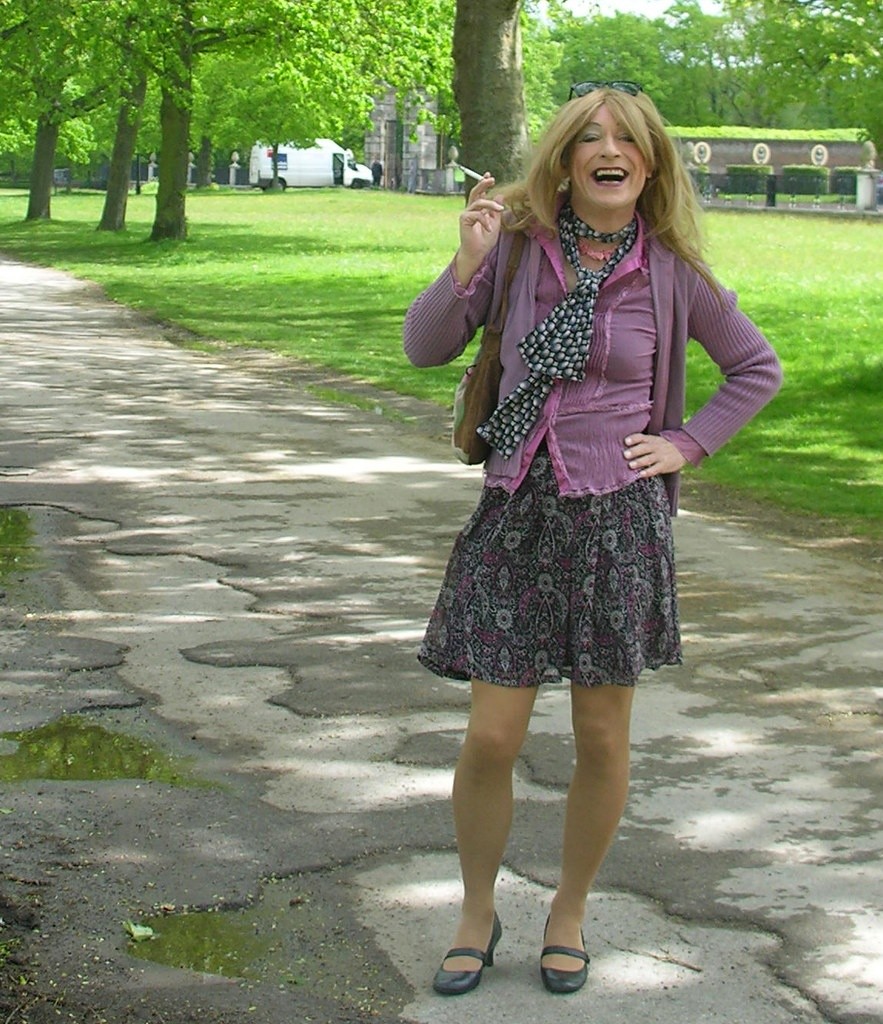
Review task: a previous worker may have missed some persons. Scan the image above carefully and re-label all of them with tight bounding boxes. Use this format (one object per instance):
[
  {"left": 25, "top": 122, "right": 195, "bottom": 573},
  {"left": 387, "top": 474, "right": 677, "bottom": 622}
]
[
  {"left": 371, "top": 160, "right": 382, "bottom": 189},
  {"left": 401, "top": 79, "right": 782, "bottom": 996}
]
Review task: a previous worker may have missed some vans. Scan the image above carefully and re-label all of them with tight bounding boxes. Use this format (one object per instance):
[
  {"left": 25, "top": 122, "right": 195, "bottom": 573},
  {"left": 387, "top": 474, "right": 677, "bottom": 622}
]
[{"left": 247, "top": 136, "right": 375, "bottom": 193}]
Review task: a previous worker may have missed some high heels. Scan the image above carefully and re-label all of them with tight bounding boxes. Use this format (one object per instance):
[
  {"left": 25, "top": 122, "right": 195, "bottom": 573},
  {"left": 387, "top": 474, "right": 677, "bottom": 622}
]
[
  {"left": 432, "top": 911, "right": 502, "bottom": 996},
  {"left": 540, "top": 911, "right": 590, "bottom": 994}
]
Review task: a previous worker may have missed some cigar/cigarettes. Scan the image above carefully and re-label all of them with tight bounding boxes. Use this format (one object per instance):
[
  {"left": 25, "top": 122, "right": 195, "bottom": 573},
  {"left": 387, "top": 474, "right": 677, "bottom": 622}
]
[{"left": 458, "top": 164, "right": 486, "bottom": 181}]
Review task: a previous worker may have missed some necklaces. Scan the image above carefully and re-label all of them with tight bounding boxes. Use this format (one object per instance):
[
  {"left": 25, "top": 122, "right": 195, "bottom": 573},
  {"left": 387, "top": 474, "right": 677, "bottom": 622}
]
[{"left": 575, "top": 237, "right": 620, "bottom": 265}]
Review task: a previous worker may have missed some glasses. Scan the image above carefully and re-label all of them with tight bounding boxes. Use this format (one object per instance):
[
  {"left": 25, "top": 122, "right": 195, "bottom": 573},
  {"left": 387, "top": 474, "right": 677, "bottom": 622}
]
[{"left": 568, "top": 80, "right": 644, "bottom": 101}]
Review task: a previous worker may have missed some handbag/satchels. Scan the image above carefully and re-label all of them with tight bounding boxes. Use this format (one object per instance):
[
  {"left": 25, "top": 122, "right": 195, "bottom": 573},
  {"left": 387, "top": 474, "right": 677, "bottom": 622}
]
[{"left": 452, "top": 230, "right": 527, "bottom": 465}]
